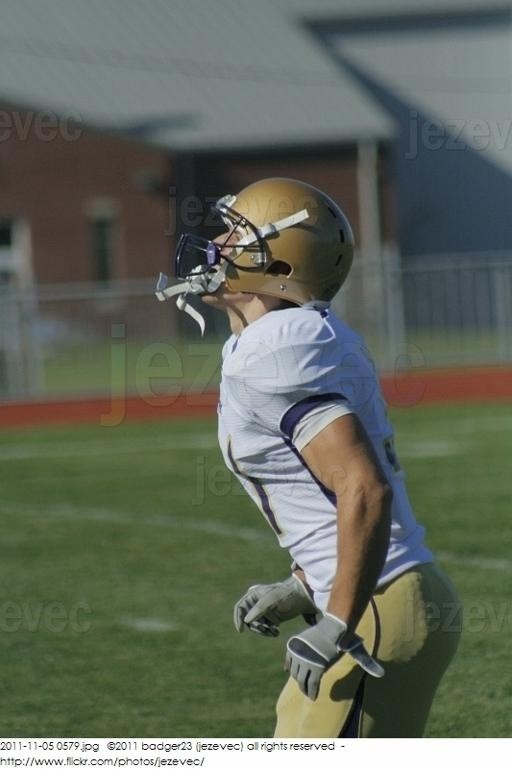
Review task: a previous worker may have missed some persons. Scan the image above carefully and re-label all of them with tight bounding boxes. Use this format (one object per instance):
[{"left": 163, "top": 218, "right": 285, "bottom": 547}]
[{"left": 156, "top": 177, "right": 463, "bottom": 738}]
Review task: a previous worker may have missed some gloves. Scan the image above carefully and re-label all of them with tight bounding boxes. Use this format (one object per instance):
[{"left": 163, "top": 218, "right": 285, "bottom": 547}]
[
  {"left": 284, "top": 613, "right": 385, "bottom": 702},
  {"left": 233, "top": 573, "right": 321, "bottom": 637}
]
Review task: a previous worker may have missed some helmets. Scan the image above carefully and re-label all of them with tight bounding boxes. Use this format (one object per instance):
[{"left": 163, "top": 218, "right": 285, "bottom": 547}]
[{"left": 155, "top": 178, "right": 355, "bottom": 337}]
